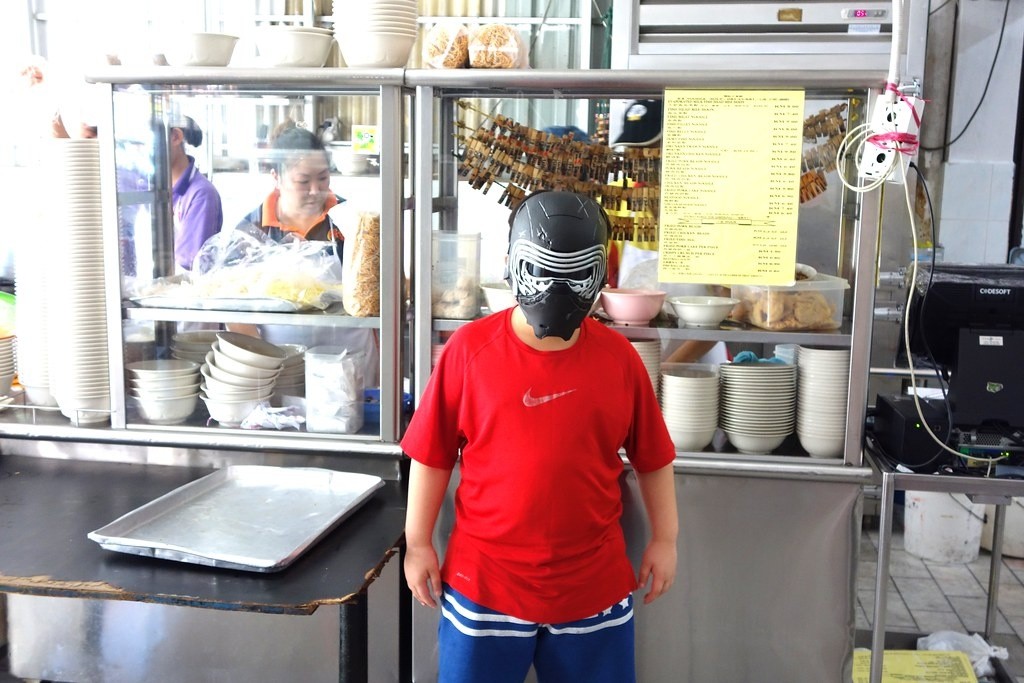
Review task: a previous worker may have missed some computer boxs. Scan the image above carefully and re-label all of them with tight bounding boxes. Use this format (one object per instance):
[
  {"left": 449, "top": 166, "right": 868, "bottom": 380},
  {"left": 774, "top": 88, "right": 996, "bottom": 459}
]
[{"left": 874, "top": 394, "right": 1024, "bottom": 481}]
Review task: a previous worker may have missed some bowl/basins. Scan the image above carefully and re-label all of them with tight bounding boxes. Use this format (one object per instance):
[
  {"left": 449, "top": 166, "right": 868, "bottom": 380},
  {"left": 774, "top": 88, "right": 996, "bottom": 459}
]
[
  {"left": 0, "top": 139, "right": 110, "bottom": 424},
  {"left": 794, "top": 263, "right": 818, "bottom": 280},
  {"left": 602, "top": 287, "right": 666, "bottom": 326},
  {"left": 432, "top": 342, "right": 445, "bottom": 373},
  {"left": 728, "top": 272, "right": 851, "bottom": 333},
  {"left": 479, "top": 282, "right": 518, "bottom": 313},
  {"left": 252, "top": 0, "right": 419, "bottom": 68},
  {"left": 629, "top": 340, "right": 850, "bottom": 459},
  {"left": 170, "top": 32, "right": 239, "bottom": 66},
  {"left": 666, "top": 294, "right": 742, "bottom": 326},
  {"left": 122, "top": 318, "right": 307, "bottom": 428},
  {"left": 331, "top": 145, "right": 368, "bottom": 177}
]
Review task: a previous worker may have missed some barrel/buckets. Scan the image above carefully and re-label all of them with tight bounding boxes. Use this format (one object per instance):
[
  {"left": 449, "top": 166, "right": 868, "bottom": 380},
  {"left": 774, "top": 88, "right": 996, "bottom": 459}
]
[
  {"left": 980, "top": 496, "right": 1024, "bottom": 559},
  {"left": 904, "top": 490, "right": 984, "bottom": 565}
]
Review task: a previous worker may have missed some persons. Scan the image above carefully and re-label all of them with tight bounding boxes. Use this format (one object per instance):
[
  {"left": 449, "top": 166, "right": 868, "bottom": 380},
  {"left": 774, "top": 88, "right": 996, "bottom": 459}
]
[
  {"left": 400, "top": 190, "right": 680, "bottom": 683},
  {"left": 605, "top": 98, "right": 731, "bottom": 365},
  {"left": 227, "top": 117, "right": 380, "bottom": 388},
  {"left": 51, "top": 113, "right": 220, "bottom": 332}
]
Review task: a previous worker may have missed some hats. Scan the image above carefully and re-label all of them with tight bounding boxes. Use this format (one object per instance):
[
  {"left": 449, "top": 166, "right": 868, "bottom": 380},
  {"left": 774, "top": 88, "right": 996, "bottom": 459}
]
[{"left": 611, "top": 99, "right": 661, "bottom": 148}]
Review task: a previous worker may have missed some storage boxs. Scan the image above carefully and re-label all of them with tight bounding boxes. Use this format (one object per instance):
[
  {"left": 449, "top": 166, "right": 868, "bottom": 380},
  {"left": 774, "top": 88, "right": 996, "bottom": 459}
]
[
  {"left": 730, "top": 267, "right": 850, "bottom": 331},
  {"left": 302, "top": 346, "right": 366, "bottom": 435},
  {"left": 430, "top": 229, "right": 482, "bottom": 320}
]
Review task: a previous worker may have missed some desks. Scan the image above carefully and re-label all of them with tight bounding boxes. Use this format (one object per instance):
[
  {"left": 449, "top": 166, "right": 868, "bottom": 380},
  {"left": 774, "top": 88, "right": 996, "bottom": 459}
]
[
  {"left": 858, "top": 406, "right": 1024, "bottom": 683},
  {"left": 0, "top": 453, "right": 405, "bottom": 683}
]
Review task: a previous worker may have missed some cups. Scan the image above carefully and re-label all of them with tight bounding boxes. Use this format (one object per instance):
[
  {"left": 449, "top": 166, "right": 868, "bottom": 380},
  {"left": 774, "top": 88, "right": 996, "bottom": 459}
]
[{"left": 432, "top": 228, "right": 482, "bottom": 320}]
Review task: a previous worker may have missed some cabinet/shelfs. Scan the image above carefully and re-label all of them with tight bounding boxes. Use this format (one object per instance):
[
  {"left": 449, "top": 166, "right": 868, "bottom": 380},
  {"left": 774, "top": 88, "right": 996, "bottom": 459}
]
[{"left": 0, "top": 67, "right": 888, "bottom": 683}]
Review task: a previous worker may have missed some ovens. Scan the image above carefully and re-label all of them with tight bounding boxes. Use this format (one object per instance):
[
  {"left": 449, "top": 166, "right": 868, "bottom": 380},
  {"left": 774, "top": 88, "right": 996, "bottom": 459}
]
[{"left": 606, "top": 0, "right": 925, "bottom": 525}]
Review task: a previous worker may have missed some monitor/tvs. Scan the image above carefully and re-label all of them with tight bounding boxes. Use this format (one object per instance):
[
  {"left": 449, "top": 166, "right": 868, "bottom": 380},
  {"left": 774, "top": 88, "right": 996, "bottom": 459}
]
[{"left": 893, "top": 260, "right": 1024, "bottom": 432}]
